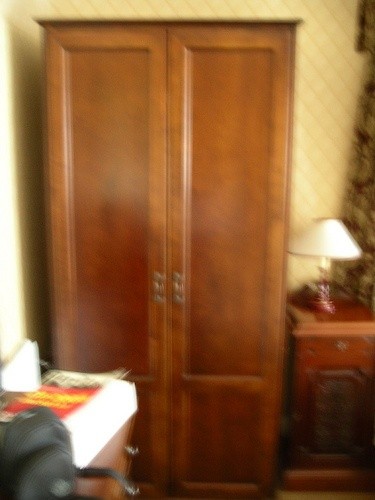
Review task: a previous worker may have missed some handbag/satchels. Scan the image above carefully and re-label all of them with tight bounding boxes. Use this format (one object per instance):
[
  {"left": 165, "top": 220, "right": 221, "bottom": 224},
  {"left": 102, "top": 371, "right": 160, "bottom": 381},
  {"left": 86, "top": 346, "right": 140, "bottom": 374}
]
[{"left": 1, "top": 406, "right": 74, "bottom": 500}]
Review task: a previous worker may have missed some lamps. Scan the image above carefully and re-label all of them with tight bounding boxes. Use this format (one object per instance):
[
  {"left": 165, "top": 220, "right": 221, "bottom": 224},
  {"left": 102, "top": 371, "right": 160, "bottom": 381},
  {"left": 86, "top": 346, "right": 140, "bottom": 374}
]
[{"left": 286, "top": 217, "right": 362, "bottom": 313}]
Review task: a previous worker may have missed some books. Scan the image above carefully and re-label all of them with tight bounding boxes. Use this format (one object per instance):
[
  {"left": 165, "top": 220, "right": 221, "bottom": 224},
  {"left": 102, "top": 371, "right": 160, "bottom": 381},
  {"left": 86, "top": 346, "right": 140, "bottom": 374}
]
[{"left": 0, "top": 370, "right": 140, "bottom": 468}]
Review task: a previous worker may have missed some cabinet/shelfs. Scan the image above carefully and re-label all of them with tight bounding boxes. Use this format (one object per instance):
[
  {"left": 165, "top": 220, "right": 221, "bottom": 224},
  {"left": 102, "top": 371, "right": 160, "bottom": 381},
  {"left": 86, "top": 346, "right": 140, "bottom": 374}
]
[
  {"left": 37, "top": 18, "right": 301, "bottom": 500},
  {"left": 284, "top": 294, "right": 375, "bottom": 491}
]
